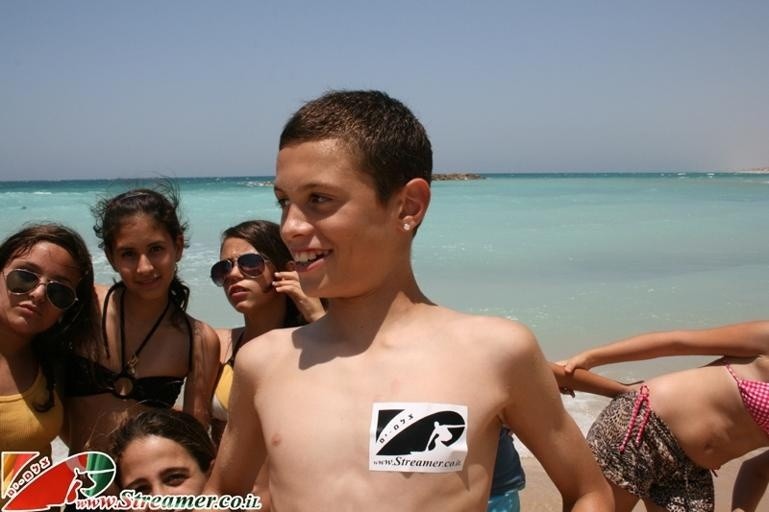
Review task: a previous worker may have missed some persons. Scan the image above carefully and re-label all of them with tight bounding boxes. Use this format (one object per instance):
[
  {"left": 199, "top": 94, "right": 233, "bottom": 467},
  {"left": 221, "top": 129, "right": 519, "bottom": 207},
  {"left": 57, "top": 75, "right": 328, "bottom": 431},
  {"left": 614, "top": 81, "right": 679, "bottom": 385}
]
[
  {"left": 483, "top": 360, "right": 643, "bottom": 511},
  {"left": 0, "top": 223, "right": 108, "bottom": 511},
  {"left": 48, "top": 177, "right": 222, "bottom": 510},
  {"left": 104, "top": 395, "right": 218, "bottom": 511},
  {"left": 210, "top": 216, "right": 326, "bottom": 512},
  {"left": 192, "top": 89, "right": 617, "bottom": 512},
  {"left": 554, "top": 319, "right": 768, "bottom": 511}
]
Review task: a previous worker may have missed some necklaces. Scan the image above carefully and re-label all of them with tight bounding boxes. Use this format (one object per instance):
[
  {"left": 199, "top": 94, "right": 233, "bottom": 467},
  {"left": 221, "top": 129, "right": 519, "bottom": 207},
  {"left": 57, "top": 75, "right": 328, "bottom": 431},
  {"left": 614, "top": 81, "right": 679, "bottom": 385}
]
[{"left": 122, "top": 294, "right": 172, "bottom": 369}]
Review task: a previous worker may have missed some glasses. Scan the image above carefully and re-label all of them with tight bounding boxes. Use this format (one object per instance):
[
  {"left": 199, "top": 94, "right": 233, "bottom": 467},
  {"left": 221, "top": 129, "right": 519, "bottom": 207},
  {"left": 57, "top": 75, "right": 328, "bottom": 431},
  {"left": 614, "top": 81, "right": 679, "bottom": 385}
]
[
  {"left": 209, "top": 252, "right": 280, "bottom": 288},
  {"left": 2, "top": 266, "right": 80, "bottom": 313}
]
